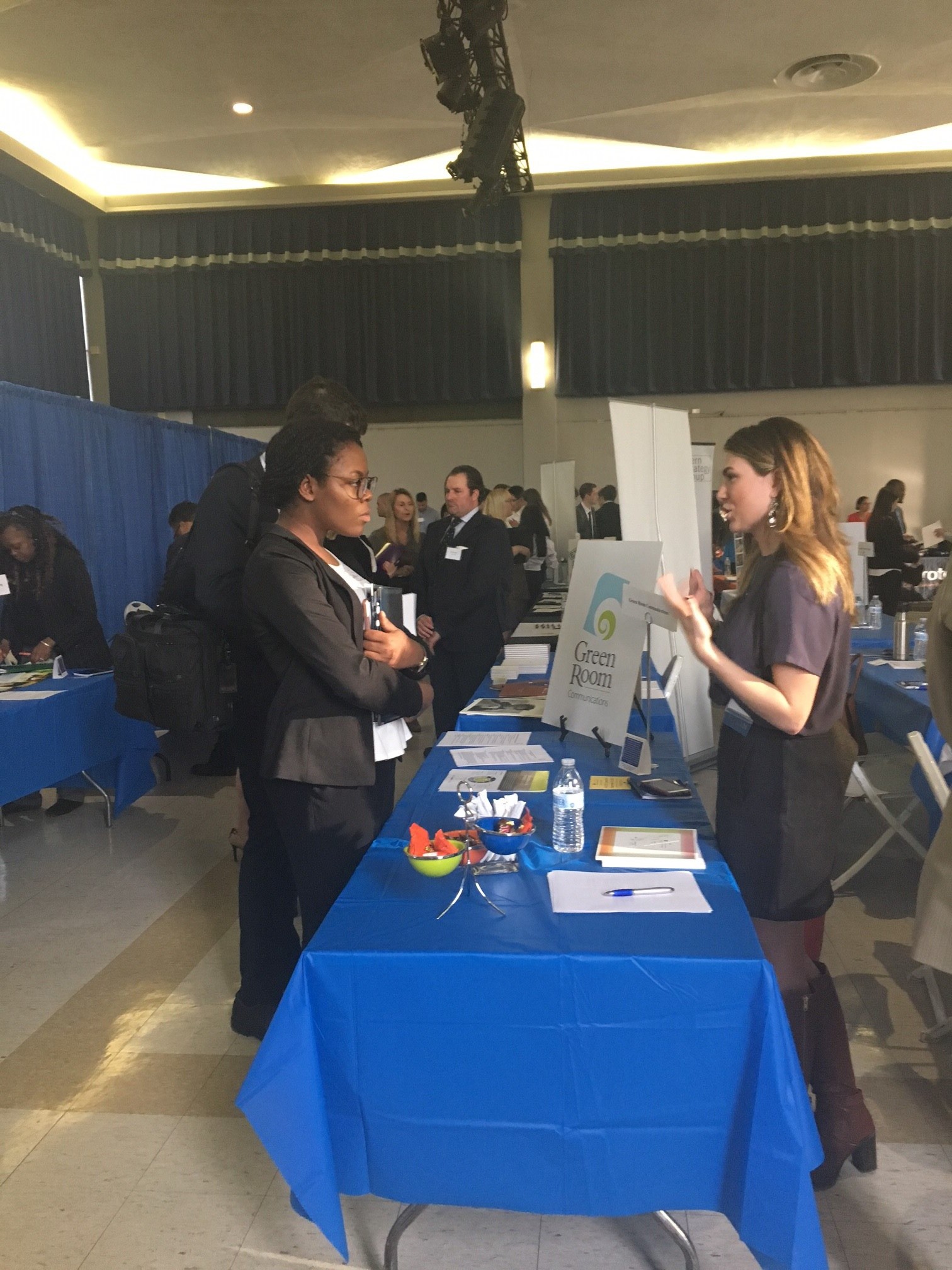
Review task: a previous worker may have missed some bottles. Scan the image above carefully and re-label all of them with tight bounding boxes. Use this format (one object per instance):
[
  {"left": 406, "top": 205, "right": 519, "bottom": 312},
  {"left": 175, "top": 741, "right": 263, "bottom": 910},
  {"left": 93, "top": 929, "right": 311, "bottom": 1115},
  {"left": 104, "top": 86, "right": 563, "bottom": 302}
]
[
  {"left": 869, "top": 595, "right": 882, "bottom": 629},
  {"left": 724, "top": 557, "right": 731, "bottom": 577},
  {"left": 551, "top": 759, "right": 585, "bottom": 854},
  {"left": 854, "top": 596, "right": 865, "bottom": 624},
  {"left": 912, "top": 618, "right": 928, "bottom": 661}
]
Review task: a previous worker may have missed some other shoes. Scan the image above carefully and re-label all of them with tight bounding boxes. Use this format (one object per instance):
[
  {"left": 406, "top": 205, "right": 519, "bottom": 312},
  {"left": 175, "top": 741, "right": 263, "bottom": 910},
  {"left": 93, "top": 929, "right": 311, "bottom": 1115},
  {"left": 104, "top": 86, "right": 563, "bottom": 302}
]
[
  {"left": 231, "top": 993, "right": 280, "bottom": 1041},
  {"left": 423, "top": 747, "right": 432, "bottom": 757},
  {"left": 190, "top": 762, "right": 236, "bottom": 777},
  {"left": 407, "top": 719, "right": 421, "bottom": 733},
  {"left": 45, "top": 798, "right": 77, "bottom": 817},
  {"left": 2, "top": 794, "right": 43, "bottom": 814}
]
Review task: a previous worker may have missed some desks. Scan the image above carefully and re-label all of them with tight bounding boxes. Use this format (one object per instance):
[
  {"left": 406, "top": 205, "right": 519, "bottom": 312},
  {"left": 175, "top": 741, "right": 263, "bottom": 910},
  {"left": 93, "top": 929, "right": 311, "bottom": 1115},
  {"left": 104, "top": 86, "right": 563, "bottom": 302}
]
[
  {"left": 232, "top": 546, "right": 952, "bottom": 1269},
  {"left": 0, "top": 668, "right": 164, "bottom": 827}
]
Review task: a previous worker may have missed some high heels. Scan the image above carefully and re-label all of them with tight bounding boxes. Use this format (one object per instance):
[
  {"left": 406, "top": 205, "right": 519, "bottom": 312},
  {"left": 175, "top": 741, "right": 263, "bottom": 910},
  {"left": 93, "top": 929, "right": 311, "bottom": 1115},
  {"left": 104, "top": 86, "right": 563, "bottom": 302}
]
[
  {"left": 229, "top": 828, "right": 244, "bottom": 863},
  {"left": 779, "top": 961, "right": 878, "bottom": 1192}
]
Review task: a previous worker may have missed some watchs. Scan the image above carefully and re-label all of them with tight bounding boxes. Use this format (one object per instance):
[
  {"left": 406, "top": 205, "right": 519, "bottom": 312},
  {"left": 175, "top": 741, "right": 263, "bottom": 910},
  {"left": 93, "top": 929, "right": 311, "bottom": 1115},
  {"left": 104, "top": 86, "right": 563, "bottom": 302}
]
[{"left": 407, "top": 643, "right": 429, "bottom": 675}]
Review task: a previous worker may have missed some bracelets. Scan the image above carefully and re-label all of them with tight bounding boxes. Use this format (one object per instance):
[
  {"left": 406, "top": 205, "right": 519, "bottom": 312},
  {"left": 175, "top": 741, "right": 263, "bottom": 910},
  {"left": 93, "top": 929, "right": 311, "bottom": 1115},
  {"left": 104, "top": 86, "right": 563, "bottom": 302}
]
[{"left": 40, "top": 641, "right": 54, "bottom": 649}]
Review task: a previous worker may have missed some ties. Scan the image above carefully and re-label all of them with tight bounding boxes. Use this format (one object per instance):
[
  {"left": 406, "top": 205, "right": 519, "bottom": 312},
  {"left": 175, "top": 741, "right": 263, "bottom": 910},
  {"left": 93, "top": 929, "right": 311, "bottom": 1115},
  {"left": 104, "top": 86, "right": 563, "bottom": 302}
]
[
  {"left": 435, "top": 518, "right": 463, "bottom": 572},
  {"left": 588, "top": 511, "right": 592, "bottom": 539}
]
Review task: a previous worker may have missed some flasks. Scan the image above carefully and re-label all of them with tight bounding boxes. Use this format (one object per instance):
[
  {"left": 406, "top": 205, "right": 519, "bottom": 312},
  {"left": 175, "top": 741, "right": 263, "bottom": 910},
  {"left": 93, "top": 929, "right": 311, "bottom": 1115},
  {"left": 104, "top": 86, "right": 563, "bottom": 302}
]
[{"left": 893, "top": 612, "right": 910, "bottom": 661}]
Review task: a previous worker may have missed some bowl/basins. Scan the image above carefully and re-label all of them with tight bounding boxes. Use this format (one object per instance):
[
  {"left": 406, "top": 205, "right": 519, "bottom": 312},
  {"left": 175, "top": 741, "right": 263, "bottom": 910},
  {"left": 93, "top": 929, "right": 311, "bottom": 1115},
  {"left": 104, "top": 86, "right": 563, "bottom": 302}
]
[
  {"left": 403, "top": 839, "right": 466, "bottom": 877},
  {"left": 443, "top": 829, "right": 488, "bottom": 866},
  {"left": 475, "top": 817, "right": 537, "bottom": 855}
]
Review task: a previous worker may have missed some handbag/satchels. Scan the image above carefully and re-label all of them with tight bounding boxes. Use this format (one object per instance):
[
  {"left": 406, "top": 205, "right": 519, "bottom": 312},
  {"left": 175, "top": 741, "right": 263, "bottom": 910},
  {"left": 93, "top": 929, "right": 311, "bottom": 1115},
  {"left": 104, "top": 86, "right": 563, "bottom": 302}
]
[
  {"left": 108, "top": 604, "right": 225, "bottom": 735},
  {"left": 845, "top": 653, "right": 868, "bottom": 767}
]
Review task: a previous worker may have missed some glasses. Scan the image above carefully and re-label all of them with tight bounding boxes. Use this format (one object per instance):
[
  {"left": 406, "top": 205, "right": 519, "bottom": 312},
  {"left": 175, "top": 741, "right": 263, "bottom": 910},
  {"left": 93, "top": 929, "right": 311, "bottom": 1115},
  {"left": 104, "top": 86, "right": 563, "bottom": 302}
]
[
  {"left": 321, "top": 472, "right": 377, "bottom": 500},
  {"left": 173, "top": 525, "right": 182, "bottom": 540},
  {"left": 0, "top": 510, "right": 26, "bottom": 520}
]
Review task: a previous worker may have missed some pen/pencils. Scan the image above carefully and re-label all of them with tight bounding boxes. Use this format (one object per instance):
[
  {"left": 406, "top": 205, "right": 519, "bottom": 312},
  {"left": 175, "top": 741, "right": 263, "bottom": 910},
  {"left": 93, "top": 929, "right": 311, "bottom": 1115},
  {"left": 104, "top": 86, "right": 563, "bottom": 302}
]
[
  {"left": 548, "top": 593, "right": 561, "bottom": 595},
  {"left": 395, "top": 560, "right": 400, "bottom": 566},
  {"left": 19, "top": 653, "right": 31, "bottom": 655},
  {"left": 602, "top": 887, "right": 675, "bottom": 896},
  {"left": 376, "top": 602, "right": 379, "bottom": 630}
]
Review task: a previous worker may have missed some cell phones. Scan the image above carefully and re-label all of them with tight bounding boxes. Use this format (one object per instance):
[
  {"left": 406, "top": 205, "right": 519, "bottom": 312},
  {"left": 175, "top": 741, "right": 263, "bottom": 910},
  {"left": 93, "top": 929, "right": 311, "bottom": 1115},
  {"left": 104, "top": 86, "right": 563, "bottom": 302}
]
[{"left": 641, "top": 778, "right": 689, "bottom": 796}]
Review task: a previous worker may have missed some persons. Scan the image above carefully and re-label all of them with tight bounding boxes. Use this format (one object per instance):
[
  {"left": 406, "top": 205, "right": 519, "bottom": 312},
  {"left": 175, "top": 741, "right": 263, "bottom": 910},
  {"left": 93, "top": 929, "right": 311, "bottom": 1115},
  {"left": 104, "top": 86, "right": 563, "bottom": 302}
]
[
  {"left": 0, "top": 375, "right": 551, "bottom": 861},
  {"left": 656, "top": 416, "right": 876, "bottom": 1193},
  {"left": 176, "top": 378, "right": 368, "bottom": 1038},
  {"left": 848, "top": 479, "right": 952, "bottom": 979},
  {"left": 229, "top": 412, "right": 436, "bottom": 946},
  {"left": 575, "top": 483, "right": 621, "bottom": 541}
]
